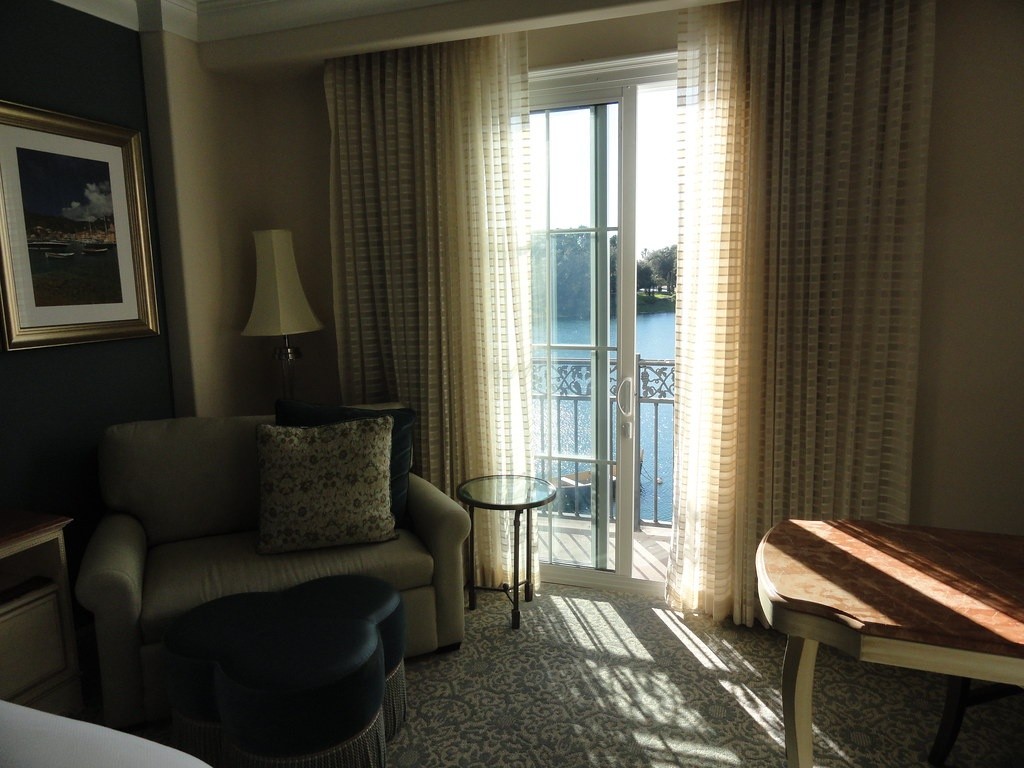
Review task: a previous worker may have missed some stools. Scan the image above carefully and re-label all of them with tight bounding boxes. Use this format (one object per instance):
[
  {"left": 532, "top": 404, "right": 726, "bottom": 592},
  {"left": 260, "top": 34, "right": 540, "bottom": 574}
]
[{"left": 172, "top": 564, "right": 408, "bottom": 768}]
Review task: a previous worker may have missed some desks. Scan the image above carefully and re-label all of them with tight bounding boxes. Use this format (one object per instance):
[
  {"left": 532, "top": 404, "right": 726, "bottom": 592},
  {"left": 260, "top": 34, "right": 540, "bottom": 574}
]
[
  {"left": 456, "top": 473, "right": 557, "bottom": 630},
  {"left": 754, "top": 516, "right": 1024, "bottom": 768}
]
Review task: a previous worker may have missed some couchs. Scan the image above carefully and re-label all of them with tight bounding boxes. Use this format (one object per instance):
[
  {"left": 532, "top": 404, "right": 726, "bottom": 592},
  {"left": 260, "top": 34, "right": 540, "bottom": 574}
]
[{"left": 72, "top": 403, "right": 472, "bottom": 729}]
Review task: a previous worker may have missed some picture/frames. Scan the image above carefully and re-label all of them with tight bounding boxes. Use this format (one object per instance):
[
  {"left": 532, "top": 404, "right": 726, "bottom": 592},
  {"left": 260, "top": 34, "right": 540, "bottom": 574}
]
[{"left": 0, "top": 96, "right": 163, "bottom": 352}]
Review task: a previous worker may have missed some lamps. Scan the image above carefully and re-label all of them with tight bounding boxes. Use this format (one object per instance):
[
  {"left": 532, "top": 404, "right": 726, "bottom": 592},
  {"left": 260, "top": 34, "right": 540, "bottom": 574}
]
[{"left": 243, "top": 229, "right": 324, "bottom": 421}]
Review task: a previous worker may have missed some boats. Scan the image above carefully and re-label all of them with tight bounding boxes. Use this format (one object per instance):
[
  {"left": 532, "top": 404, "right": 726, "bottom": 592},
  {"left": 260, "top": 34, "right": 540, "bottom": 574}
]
[
  {"left": 44, "top": 251, "right": 75, "bottom": 259},
  {"left": 533, "top": 447, "right": 644, "bottom": 509}
]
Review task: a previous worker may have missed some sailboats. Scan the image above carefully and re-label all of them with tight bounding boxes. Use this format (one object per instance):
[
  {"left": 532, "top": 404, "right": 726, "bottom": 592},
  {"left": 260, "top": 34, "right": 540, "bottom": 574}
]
[{"left": 81, "top": 204, "right": 110, "bottom": 255}]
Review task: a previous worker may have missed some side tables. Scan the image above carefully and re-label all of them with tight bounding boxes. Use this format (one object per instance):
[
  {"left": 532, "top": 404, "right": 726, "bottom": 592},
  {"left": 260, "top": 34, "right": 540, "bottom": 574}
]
[{"left": 0, "top": 485, "right": 99, "bottom": 728}]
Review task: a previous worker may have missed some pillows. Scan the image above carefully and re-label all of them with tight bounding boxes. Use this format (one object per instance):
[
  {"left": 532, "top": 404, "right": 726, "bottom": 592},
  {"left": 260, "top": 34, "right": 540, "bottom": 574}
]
[
  {"left": 255, "top": 418, "right": 400, "bottom": 551},
  {"left": 279, "top": 404, "right": 417, "bottom": 532}
]
